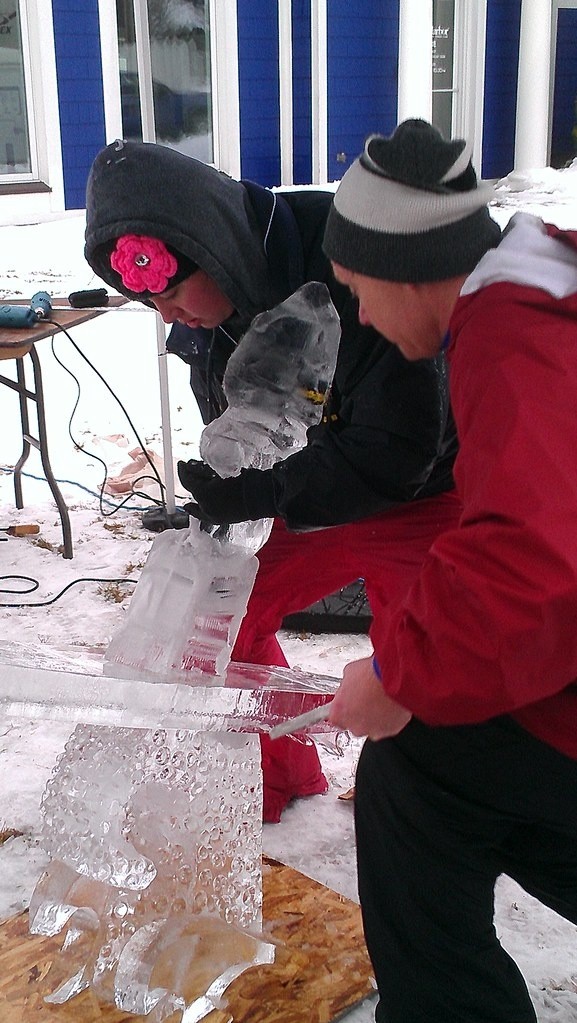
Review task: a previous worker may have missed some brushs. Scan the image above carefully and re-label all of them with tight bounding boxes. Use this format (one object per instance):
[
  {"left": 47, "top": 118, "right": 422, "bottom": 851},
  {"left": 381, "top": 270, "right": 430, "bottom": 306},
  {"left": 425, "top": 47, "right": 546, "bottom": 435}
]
[{"left": 68, "top": 288, "right": 108, "bottom": 308}]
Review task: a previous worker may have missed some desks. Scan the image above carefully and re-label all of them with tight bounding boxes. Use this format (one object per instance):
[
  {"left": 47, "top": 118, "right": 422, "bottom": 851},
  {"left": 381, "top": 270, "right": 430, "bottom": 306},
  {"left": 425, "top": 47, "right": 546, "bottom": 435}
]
[{"left": 0, "top": 296, "right": 176, "bottom": 560}]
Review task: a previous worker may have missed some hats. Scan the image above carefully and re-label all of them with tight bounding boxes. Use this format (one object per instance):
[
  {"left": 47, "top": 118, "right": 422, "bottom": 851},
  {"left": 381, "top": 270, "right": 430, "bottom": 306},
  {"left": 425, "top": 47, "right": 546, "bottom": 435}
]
[
  {"left": 99, "top": 234, "right": 199, "bottom": 301},
  {"left": 323, "top": 120, "right": 503, "bottom": 284}
]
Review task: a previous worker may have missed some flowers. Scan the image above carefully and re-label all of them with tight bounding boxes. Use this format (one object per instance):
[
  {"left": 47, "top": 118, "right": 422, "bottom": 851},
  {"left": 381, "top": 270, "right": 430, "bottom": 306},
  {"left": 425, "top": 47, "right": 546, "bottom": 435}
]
[{"left": 111, "top": 235, "right": 178, "bottom": 293}]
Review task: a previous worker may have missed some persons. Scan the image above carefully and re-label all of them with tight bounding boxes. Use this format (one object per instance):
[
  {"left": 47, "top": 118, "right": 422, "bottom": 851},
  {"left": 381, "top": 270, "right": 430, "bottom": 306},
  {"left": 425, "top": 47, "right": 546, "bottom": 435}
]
[
  {"left": 83, "top": 140, "right": 459, "bottom": 829},
  {"left": 172, "top": 118, "right": 577, "bottom": 1023}
]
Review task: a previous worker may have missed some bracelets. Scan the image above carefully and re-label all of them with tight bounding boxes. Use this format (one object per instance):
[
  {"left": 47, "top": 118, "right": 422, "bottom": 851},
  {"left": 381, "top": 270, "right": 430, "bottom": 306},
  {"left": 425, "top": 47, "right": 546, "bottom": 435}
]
[{"left": 371, "top": 657, "right": 380, "bottom": 680}]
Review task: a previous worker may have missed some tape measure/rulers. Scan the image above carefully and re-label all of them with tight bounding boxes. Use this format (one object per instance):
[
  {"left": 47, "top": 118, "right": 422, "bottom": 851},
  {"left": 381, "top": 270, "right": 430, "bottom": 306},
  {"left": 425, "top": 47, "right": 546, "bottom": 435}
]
[{"left": 12, "top": 305, "right": 153, "bottom": 311}]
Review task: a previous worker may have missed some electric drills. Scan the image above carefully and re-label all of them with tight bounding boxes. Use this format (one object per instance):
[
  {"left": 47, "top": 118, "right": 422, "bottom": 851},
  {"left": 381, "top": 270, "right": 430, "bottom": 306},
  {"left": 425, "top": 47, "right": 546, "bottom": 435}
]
[
  {"left": 0, "top": 303, "right": 38, "bottom": 328},
  {"left": 31, "top": 290, "right": 52, "bottom": 317}
]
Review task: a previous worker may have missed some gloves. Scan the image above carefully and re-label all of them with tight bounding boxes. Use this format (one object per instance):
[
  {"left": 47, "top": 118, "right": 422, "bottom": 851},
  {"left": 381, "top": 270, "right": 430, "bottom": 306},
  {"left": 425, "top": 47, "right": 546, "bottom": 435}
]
[{"left": 177, "top": 458, "right": 280, "bottom": 526}]
